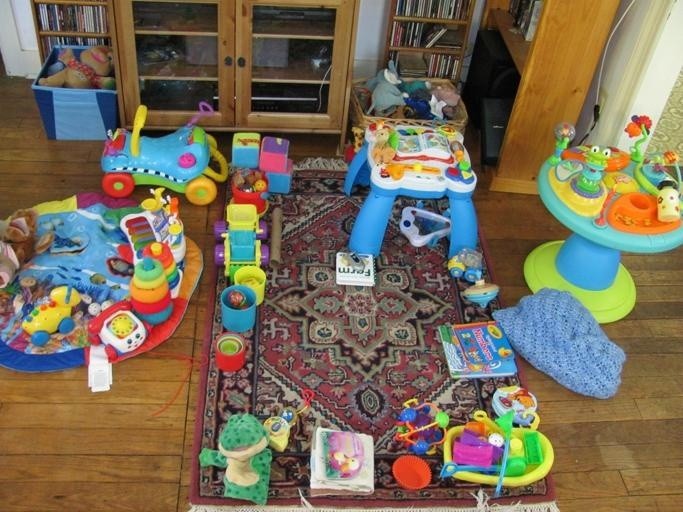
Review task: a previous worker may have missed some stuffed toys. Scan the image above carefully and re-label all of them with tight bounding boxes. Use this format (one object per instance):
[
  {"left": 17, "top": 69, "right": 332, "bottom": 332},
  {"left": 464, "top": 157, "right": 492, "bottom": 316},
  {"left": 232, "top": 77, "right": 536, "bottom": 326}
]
[
  {"left": 0, "top": 209, "right": 54, "bottom": 287},
  {"left": 359, "top": 60, "right": 462, "bottom": 121},
  {"left": 197, "top": 412, "right": 270, "bottom": 506},
  {"left": 322, "top": 432, "right": 365, "bottom": 480},
  {"left": 34, "top": 45, "right": 114, "bottom": 90}
]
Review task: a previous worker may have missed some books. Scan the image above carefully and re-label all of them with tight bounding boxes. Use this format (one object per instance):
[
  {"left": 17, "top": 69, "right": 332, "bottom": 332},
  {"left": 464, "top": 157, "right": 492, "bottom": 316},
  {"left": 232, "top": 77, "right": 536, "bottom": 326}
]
[
  {"left": 387, "top": 0, "right": 469, "bottom": 85},
  {"left": 331, "top": 251, "right": 373, "bottom": 286},
  {"left": 451, "top": 322, "right": 515, "bottom": 367},
  {"left": 307, "top": 428, "right": 374, "bottom": 497},
  {"left": 435, "top": 321, "right": 515, "bottom": 379},
  {"left": 507, "top": 0, "right": 543, "bottom": 43},
  {"left": 33, "top": 2, "right": 110, "bottom": 63}
]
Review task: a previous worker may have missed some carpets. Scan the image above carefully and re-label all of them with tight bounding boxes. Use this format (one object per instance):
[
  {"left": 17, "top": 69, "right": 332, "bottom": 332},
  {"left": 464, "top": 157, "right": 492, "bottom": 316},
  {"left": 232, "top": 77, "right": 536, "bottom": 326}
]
[{"left": 185, "top": 156, "right": 559, "bottom": 512}]
